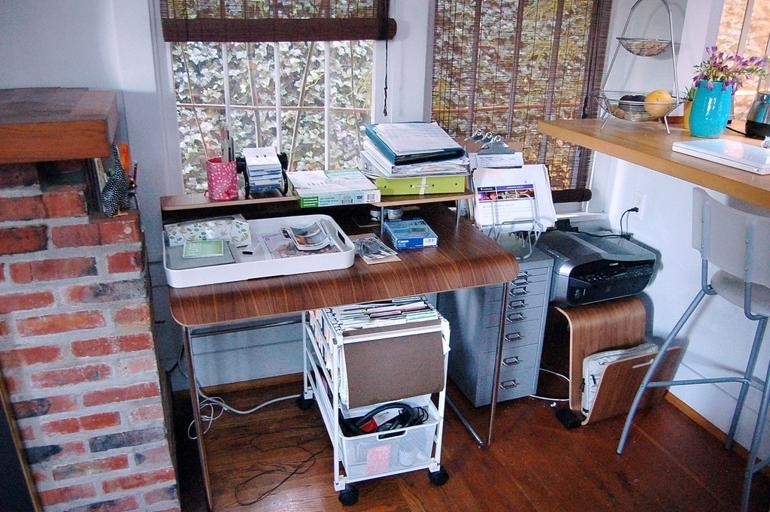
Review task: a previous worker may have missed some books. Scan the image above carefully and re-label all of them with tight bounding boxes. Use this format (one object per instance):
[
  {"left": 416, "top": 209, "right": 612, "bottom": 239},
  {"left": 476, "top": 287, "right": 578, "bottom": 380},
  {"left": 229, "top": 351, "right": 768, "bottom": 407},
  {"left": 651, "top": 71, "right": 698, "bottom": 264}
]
[
  {"left": 310, "top": 296, "right": 444, "bottom": 409},
  {"left": 286, "top": 222, "right": 332, "bottom": 251},
  {"left": 467, "top": 152, "right": 557, "bottom": 231},
  {"left": 242, "top": 146, "right": 285, "bottom": 194}
]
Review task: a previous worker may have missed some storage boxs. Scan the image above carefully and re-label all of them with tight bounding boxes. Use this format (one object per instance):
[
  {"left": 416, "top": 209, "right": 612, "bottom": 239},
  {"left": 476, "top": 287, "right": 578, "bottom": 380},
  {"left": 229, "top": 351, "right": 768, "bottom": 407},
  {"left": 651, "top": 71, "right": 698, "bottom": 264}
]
[{"left": 313, "top": 376, "right": 443, "bottom": 481}]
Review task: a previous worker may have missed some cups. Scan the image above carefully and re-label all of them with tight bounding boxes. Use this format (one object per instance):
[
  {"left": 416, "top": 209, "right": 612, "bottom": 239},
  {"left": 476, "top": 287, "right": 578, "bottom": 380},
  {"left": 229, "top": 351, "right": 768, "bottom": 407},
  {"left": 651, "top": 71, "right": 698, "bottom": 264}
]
[{"left": 205, "top": 156, "right": 238, "bottom": 201}]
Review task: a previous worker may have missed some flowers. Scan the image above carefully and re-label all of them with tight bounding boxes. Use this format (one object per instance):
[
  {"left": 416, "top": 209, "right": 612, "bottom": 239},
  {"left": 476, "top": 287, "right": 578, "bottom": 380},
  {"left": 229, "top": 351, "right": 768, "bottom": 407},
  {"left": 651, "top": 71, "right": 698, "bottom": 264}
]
[{"left": 683, "top": 42, "right": 769, "bottom": 93}]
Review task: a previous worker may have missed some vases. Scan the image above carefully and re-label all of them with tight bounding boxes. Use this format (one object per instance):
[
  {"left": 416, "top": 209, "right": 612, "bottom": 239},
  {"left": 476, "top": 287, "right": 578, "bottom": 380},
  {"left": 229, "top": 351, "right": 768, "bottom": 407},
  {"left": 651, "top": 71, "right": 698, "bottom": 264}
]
[{"left": 687, "top": 75, "right": 731, "bottom": 139}]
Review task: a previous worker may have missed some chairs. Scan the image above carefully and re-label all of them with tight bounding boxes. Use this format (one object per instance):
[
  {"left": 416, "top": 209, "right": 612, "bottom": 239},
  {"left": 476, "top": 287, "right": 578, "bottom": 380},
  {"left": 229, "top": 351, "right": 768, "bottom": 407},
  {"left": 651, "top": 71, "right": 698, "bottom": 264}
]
[{"left": 617, "top": 182, "right": 769, "bottom": 510}]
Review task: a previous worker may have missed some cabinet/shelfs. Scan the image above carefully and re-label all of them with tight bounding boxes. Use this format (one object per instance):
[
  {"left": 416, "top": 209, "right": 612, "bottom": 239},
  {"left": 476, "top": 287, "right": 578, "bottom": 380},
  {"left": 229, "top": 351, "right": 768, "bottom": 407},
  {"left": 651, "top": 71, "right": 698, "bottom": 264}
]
[
  {"left": 295, "top": 296, "right": 455, "bottom": 507},
  {"left": 440, "top": 223, "right": 555, "bottom": 414}
]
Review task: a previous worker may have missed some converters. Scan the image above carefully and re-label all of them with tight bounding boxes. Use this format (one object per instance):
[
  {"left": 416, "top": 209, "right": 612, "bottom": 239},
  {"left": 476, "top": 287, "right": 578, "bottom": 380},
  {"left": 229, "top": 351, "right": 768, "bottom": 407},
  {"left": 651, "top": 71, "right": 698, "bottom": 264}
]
[{"left": 557, "top": 408, "right": 581, "bottom": 430}]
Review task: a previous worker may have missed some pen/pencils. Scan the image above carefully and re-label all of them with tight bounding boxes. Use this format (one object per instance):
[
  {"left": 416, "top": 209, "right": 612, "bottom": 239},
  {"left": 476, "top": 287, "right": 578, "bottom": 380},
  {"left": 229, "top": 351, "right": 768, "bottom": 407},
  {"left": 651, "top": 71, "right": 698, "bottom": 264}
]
[{"left": 219, "top": 126, "right": 234, "bottom": 163}]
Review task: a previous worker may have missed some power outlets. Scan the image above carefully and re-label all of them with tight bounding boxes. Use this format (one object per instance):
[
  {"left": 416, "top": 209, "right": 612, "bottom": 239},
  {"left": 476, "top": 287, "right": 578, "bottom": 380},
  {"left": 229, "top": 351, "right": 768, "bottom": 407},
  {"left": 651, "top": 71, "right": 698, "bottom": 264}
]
[{"left": 631, "top": 190, "right": 647, "bottom": 220}]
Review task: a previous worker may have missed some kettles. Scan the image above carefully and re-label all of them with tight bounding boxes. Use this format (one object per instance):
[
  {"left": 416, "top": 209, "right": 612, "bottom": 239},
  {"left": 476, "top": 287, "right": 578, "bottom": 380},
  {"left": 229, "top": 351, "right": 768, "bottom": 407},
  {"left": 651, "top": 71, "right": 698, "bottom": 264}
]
[{"left": 746, "top": 93, "right": 770, "bottom": 140}]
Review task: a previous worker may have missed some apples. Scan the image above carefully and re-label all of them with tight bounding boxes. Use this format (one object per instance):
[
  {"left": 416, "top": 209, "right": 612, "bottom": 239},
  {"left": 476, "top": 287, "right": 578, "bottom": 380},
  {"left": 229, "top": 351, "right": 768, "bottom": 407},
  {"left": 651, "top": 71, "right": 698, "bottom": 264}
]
[{"left": 643, "top": 90, "right": 673, "bottom": 116}]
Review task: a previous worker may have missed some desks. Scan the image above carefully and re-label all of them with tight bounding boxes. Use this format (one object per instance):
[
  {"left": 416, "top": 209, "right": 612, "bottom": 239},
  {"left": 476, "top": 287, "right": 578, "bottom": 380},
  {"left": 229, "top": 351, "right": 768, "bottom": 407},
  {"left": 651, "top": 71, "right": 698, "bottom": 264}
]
[
  {"left": 159, "top": 181, "right": 522, "bottom": 510},
  {"left": 552, "top": 298, "right": 683, "bottom": 427},
  {"left": 533, "top": 110, "right": 768, "bottom": 475}
]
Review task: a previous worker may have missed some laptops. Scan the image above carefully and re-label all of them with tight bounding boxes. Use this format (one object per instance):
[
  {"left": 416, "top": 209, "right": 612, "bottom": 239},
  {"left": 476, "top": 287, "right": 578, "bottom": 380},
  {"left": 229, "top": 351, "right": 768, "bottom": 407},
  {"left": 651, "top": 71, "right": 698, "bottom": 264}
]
[{"left": 673, "top": 138, "right": 770, "bottom": 175}]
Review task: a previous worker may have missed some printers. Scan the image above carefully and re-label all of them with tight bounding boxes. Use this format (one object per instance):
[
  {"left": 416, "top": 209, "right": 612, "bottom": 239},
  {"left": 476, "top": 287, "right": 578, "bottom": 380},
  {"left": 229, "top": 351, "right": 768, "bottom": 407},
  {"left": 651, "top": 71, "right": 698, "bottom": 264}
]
[{"left": 527, "top": 229, "right": 657, "bottom": 307}]
